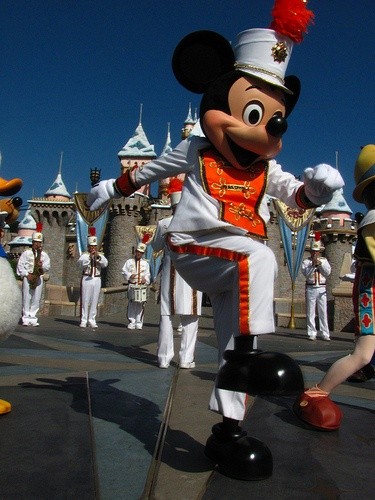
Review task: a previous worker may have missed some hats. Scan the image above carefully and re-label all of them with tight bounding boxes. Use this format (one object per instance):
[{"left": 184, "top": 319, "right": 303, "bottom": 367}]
[
  {"left": 167, "top": 177, "right": 182, "bottom": 206},
  {"left": 136, "top": 234, "right": 149, "bottom": 253},
  {"left": 31, "top": 222, "right": 43, "bottom": 242},
  {"left": 88, "top": 228, "right": 98, "bottom": 245},
  {"left": 310, "top": 232, "right": 321, "bottom": 251}
]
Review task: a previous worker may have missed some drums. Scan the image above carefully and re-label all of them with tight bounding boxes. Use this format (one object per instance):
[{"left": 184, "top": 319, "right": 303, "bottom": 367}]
[{"left": 129, "top": 285, "right": 148, "bottom": 303}]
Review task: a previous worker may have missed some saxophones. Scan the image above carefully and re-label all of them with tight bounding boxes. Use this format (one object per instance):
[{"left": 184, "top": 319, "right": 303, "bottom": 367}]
[{"left": 30, "top": 245, "right": 45, "bottom": 289}]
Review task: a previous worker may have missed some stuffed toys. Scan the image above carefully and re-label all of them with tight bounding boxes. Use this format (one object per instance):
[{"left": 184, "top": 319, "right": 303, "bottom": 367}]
[
  {"left": 295, "top": 144, "right": 375, "bottom": 430},
  {"left": 0, "top": 179, "right": 24, "bottom": 413},
  {"left": 86, "top": 27, "right": 346, "bottom": 482}
]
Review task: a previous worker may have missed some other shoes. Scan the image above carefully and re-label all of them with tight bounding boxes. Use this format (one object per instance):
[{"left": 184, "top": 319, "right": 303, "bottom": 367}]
[
  {"left": 22, "top": 323, "right": 28, "bottom": 326},
  {"left": 89, "top": 324, "right": 98, "bottom": 328},
  {"left": 323, "top": 337, "right": 330, "bottom": 341},
  {"left": 80, "top": 324, "right": 86, "bottom": 328},
  {"left": 136, "top": 327, "right": 142, "bottom": 330},
  {"left": 128, "top": 326, "right": 135, "bottom": 329},
  {"left": 31, "top": 322, "right": 39, "bottom": 327},
  {"left": 179, "top": 362, "right": 196, "bottom": 369},
  {"left": 309, "top": 336, "right": 316, "bottom": 341}
]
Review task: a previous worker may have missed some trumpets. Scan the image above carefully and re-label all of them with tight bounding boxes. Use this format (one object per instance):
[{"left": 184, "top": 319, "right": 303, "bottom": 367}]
[
  {"left": 92, "top": 248, "right": 102, "bottom": 263},
  {"left": 311, "top": 253, "right": 322, "bottom": 270}
]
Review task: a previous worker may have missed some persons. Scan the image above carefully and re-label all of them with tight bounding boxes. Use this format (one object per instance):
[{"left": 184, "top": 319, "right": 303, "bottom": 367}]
[
  {"left": 301, "top": 240, "right": 331, "bottom": 341},
  {"left": 150, "top": 191, "right": 203, "bottom": 369},
  {"left": 122, "top": 243, "right": 150, "bottom": 329},
  {"left": 77, "top": 236, "right": 108, "bottom": 328},
  {"left": 17, "top": 232, "right": 51, "bottom": 327}
]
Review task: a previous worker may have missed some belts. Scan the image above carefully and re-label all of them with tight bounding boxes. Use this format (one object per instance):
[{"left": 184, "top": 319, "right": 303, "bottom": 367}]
[
  {"left": 85, "top": 274, "right": 100, "bottom": 277},
  {"left": 306, "top": 283, "right": 325, "bottom": 286}
]
